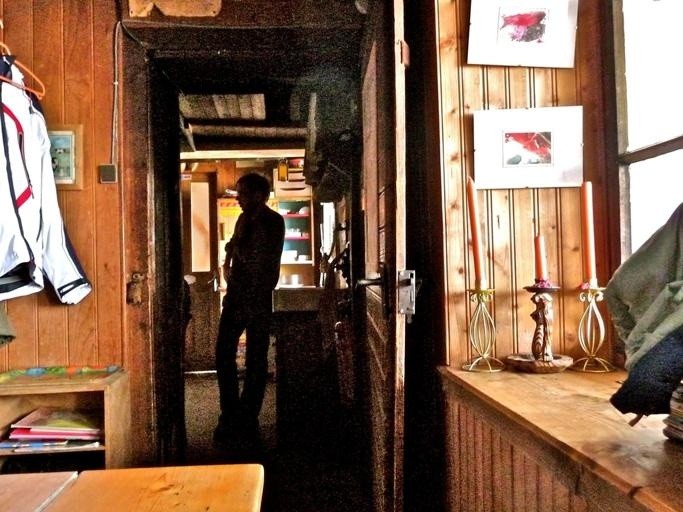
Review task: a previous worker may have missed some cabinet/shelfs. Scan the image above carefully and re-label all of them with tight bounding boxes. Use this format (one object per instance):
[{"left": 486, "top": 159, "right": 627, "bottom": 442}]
[
  {"left": 0, "top": 366, "right": 131, "bottom": 471},
  {"left": 217, "top": 191, "right": 314, "bottom": 266}
]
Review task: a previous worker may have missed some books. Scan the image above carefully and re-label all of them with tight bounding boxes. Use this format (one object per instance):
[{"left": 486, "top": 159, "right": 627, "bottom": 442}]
[{"left": 0, "top": 406, "right": 104, "bottom": 453}]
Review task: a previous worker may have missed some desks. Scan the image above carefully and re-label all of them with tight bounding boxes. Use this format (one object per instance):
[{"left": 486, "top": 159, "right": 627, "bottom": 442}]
[{"left": 2, "top": 462, "right": 266, "bottom": 511}]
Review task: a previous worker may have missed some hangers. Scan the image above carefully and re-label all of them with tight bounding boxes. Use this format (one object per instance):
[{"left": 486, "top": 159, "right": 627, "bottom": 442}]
[{"left": 0, "top": 17, "right": 49, "bottom": 102}]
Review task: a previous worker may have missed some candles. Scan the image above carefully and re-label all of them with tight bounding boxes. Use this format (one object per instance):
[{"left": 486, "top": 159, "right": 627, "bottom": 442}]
[
  {"left": 465, "top": 172, "right": 485, "bottom": 289},
  {"left": 532, "top": 233, "right": 546, "bottom": 282},
  {"left": 580, "top": 180, "right": 596, "bottom": 285}
]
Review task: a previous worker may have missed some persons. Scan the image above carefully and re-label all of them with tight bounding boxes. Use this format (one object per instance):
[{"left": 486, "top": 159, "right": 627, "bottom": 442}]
[{"left": 209, "top": 173, "right": 284, "bottom": 446}]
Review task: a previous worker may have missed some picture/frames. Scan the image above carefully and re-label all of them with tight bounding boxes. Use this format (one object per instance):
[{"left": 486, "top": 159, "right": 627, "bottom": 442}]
[
  {"left": 469, "top": 102, "right": 586, "bottom": 188},
  {"left": 463, "top": 0, "right": 580, "bottom": 70},
  {"left": 46, "top": 122, "right": 85, "bottom": 191}
]
